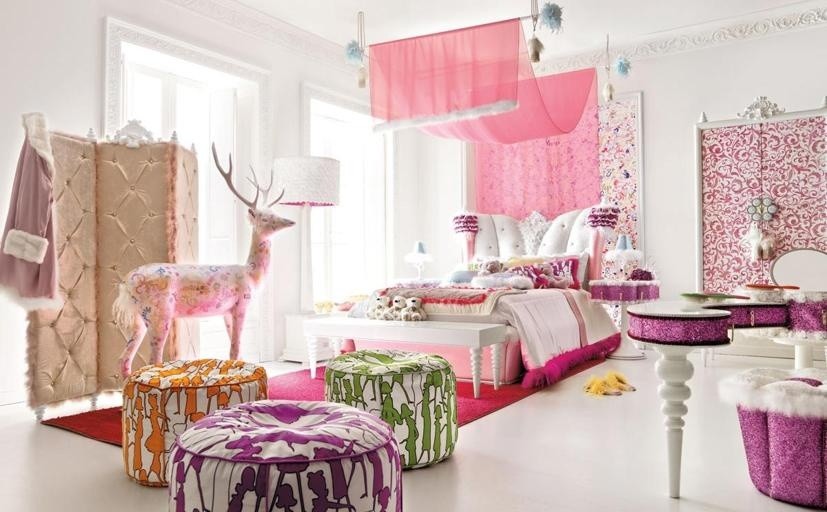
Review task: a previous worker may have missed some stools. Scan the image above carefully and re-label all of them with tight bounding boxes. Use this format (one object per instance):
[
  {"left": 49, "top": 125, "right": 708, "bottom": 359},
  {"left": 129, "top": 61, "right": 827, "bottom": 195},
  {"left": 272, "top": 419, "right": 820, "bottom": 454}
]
[
  {"left": 121, "top": 358, "right": 268, "bottom": 489},
  {"left": 770, "top": 290, "right": 827, "bottom": 370},
  {"left": 719, "top": 366, "right": 827, "bottom": 509},
  {"left": 324, "top": 348, "right": 459, "bottom": 469},
  {"left": 166, "top": 398, "right": 405, "bottom": 512}
]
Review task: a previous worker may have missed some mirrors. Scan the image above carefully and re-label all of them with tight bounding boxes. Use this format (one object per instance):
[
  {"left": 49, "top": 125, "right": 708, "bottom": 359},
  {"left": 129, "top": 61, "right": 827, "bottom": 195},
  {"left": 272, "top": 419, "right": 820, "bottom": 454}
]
[{"left": 771, "top": 248, "right": 827, "bottom": 291}]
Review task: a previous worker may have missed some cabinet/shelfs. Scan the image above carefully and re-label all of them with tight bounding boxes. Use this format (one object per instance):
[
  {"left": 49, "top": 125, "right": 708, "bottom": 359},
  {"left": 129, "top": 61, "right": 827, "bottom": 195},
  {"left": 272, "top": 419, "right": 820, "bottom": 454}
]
[{"left": 694, "top": 98, "right": 827, "bottom": 304}]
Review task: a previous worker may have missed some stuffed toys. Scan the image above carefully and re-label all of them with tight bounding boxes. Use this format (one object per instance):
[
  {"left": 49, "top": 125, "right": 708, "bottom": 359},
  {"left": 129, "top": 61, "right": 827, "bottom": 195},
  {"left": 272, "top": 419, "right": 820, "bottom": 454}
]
[
  {"left": 364, "top": 295, "right": 390, "bottom": 320},
  {"left": 380, "top": 296, "right": 406, "bottom": 321},
  {"left": 534, "top": 261, "right": 571, "bottom": 289},
  {"left": 475, "top": 260, "right": 503, "bottom": 278},
  {"left": 401, "top": 296, "right": 427, "bottom": 321}
]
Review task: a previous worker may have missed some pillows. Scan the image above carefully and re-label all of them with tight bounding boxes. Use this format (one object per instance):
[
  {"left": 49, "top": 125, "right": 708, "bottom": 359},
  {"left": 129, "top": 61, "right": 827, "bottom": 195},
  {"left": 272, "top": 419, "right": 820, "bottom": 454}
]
[{"left": 437, "top": 252, "right": 590, "bottom": 290}]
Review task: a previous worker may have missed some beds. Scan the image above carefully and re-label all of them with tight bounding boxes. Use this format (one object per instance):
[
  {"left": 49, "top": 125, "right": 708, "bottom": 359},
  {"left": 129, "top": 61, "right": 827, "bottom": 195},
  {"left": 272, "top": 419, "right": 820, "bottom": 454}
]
[{"left": 342, "top": 207, "right": 600, "bottom": 388}]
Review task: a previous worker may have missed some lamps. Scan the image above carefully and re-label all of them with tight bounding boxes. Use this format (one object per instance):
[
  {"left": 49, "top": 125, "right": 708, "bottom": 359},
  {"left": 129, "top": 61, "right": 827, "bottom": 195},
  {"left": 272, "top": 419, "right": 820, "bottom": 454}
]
[
  {"left": 279, "top": 156, "right": 340, "bottom": 365},
  {"left": 403, "top": 241, "right": 432, "bottom": 281},
  {"left": 603, "top": 235, "right": 641, "bottom": 278}
]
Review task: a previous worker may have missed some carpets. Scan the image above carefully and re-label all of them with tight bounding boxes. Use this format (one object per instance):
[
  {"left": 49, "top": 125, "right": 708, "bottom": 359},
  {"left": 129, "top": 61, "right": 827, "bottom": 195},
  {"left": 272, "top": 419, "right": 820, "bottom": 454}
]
[{"left": 40, "top": 352, "right": 607, "bottom": 451}]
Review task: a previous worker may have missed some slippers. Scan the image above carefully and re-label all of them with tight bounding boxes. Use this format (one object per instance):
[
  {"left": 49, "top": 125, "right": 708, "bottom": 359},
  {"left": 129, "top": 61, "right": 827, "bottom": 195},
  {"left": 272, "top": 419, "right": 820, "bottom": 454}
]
[
  {"left": 583, "top": 378, "right": 621, "bottom": 396},
  {"left": 600, "top": 370, "right": 636, "bottom": 392}
]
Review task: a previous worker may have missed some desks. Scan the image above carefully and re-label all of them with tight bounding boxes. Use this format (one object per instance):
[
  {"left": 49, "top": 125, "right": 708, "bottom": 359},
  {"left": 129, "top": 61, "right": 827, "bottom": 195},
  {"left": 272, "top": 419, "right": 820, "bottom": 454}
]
[
  {"left": 626, "top": 303, "right": 730, "bottom": 499},
  {"left": 589, "top": 278, "right": 659, "bottom": 361}
]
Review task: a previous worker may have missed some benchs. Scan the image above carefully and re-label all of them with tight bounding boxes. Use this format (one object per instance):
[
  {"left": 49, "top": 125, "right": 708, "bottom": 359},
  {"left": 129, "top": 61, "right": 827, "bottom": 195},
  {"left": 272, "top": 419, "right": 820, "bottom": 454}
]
[
  {"left": 692, "top": 299, "right": 785, "bottom": 325},
  {"left": 302, "top": 316, "right": 506, "bottom": 398}
]
